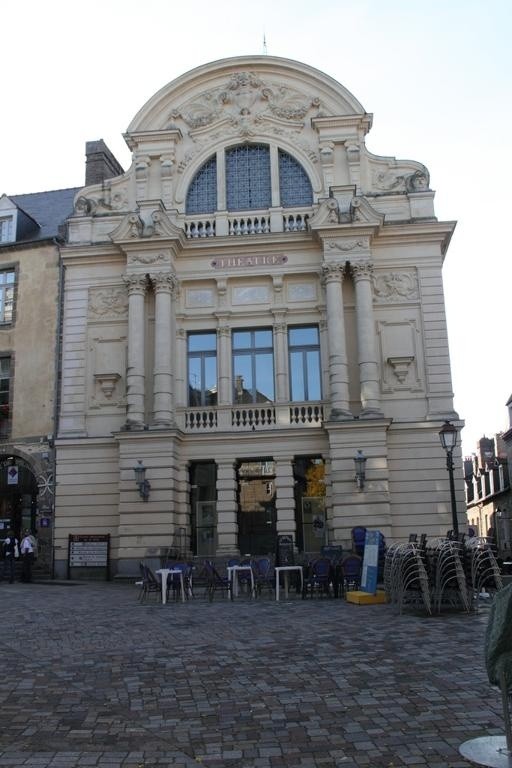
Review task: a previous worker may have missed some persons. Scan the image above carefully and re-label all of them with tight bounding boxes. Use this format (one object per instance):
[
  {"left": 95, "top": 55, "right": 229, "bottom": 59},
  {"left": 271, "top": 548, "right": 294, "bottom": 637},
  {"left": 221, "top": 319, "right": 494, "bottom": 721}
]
[
  {"left": 469, "top": 528, "right": 478, "bottom": 546},
  {"left": 0, "top": 523, "right": 39, "bottom": 584},
  {"left": 212, "top": 74, "right": 280, "bottom": 130}
]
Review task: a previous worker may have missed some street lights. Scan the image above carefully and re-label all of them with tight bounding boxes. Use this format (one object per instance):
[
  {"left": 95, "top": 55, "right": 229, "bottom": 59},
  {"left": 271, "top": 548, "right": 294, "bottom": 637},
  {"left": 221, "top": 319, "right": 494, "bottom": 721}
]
[{"left": 438, "top": 419, "right": 459, "bottom": 541}]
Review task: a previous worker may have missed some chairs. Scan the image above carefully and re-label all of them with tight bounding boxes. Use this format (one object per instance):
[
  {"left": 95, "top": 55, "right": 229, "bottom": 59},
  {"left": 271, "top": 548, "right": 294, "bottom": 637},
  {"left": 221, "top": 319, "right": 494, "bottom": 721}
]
[{"left": 136, "top": 526, "right": 506, "bottom": 617}]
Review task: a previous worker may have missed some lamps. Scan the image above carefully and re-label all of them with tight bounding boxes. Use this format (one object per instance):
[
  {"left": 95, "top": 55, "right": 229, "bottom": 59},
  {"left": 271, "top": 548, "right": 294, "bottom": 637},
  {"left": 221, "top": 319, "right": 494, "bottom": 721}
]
[
  {"left": 352, "top": 448, "right": 367, "bottom": 491},
  {"left": 132, "top": 458, "right": 150, "bottom": 503}
]
